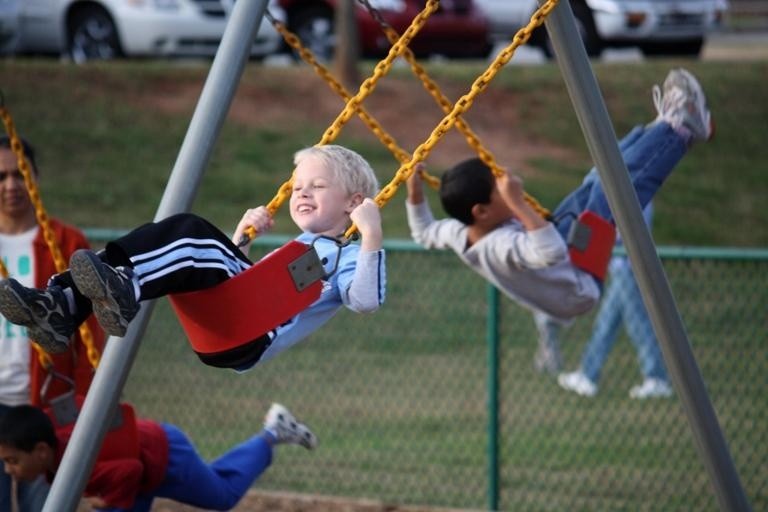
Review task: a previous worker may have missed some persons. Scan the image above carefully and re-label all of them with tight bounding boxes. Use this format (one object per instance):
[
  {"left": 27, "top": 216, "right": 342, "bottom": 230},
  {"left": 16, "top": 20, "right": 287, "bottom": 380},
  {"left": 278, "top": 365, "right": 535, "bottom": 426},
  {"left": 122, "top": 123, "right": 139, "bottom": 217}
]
[
  {"left": 557, "top": 201, "right": 674, "bottom": 399},
  {"left": 0, "top": 144, "right": 386, "bottom": 374},
  {"left": 404, "top": 69, "right": 713, "bottom": 319},
  {"left": 0, "top": 403, "right": 317, "bottom": 512},
  {"left": 0, "top": 137, "right": 108, "bottom": 512}
]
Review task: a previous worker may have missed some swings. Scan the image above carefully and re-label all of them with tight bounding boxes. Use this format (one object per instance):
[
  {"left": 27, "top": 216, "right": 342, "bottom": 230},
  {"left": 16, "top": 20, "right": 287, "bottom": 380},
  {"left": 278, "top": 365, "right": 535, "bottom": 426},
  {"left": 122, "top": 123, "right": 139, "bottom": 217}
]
[
  {"left": 0, "top": 103, "right": 145, "bottom": 511},
  {"left": 164, "top": 0, "right": 559, "bottom": 354},
  {"left": 260, "top": 1, "right": 614, "bottom": 287}
]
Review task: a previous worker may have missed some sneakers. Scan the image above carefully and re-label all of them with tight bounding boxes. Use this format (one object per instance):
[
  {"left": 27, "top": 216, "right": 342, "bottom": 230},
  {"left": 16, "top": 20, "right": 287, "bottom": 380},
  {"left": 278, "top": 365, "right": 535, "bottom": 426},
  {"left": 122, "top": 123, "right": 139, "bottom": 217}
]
[
  {"left": 655, "top": 65, "right": 715, "bottom": 144},
  {"left": 628, "top": 376, "right": 674, "bottom": 404},
  {"left": 262, "top": 402, "right": 318, "bottom": 451},
  {"left": 68, "top": 248, "right": 140, "bottom": 341},
  {"left": 556, "top": 370, "right": 599, "bottom": 400},
  {"left": 0, "top": 276, "right": 73, "bottom": 357}
]
